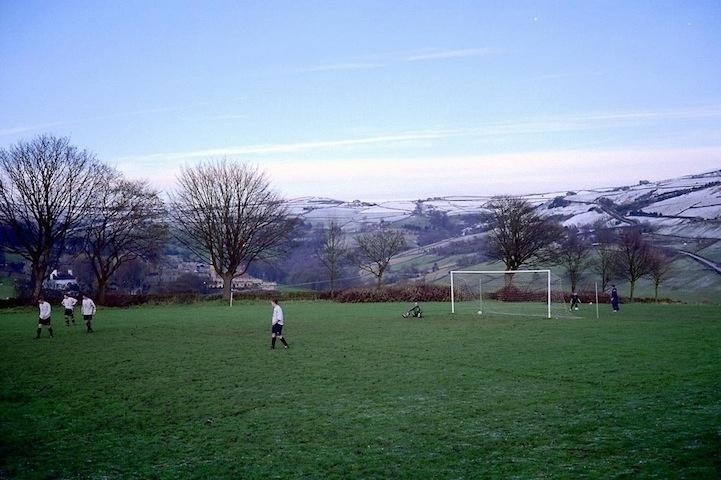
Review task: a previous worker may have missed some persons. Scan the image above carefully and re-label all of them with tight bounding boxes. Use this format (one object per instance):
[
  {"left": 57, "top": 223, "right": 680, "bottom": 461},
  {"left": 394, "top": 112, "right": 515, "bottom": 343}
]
[
  {"left": 402, "top": 302, "right": 421, "bottom": 318},
  {"left": 81, "top": 293, "right": 97, "bottom": 333},
  {"left": 61, "top": 294, "right": 78, "bottom": 328},
  {"left": 270, "top": 298, "right": 289, "bottom": 350},
  {"left": 33, "top": 297, "right": 54, "bottom": 340},
  {"left": 570, "top": 293, "right": 581, "bottom": 311},
  {"left": 610, "top": 285, "right": 619, "bottom": 312}
]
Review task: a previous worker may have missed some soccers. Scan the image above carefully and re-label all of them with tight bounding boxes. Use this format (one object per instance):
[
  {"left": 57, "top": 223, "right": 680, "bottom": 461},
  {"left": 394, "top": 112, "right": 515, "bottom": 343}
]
[
  {"left": 588, "top": 302, "right": 592, "bottom": 305},
  {"left": 574, "top": 307, "right": 578, "bottom": 310},
  {"left": 477, "top": 310, "right": 482, "bottom": 314}
]
